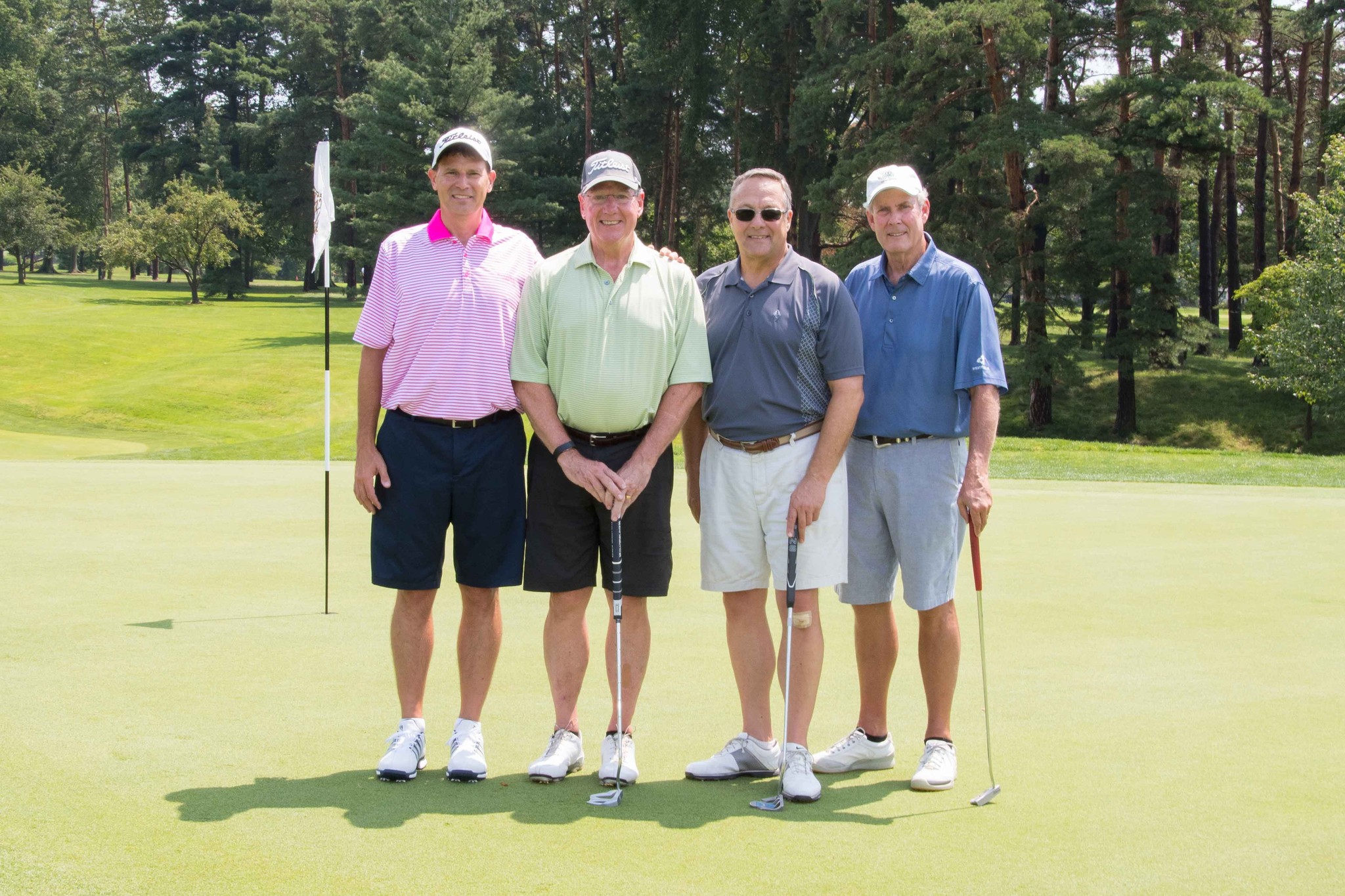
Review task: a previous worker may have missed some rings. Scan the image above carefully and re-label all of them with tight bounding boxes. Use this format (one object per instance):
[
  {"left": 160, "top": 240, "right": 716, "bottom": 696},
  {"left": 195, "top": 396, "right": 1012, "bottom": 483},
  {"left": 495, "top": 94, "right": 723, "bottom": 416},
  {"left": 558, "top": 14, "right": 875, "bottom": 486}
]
[{"left": 625, "top": 495, "right": 631, "bottom": 499}]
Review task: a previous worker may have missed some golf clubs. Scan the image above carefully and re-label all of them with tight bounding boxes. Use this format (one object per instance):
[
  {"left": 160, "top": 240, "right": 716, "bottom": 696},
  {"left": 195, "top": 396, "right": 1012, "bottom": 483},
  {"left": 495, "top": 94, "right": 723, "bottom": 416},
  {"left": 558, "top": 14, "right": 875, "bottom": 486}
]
[
  {"left": 966, "top": 509, "right": 1001, "bottom": 807},
  {"left": 748, "top": 521, "right": 805, "bottom": 813},
  {"left": 587, "top": 513, "right": 627, "bottom": 807}
]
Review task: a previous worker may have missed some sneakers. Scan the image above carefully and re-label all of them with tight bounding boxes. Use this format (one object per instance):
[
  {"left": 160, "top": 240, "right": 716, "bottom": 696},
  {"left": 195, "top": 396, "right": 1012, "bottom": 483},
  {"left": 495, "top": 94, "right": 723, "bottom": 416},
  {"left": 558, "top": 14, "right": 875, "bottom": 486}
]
[
  {"left": 376, "top": 719, "right": 427, "bottom": 782},
  {"left": 528, "top": 718, "right": 584, "bottom": 784},
  {"left": 911, "top": 739, "right": 957, "bottom": 791},
  {"left": 598, "top": 723, "right": 639, "bottom": 787},
  {"left": 777, "top": 742, "right": 822, "bottom": 802},
  {"left": 685, "top": 732, "right": 781, "bottom": 781},
  {"left": 445, "top": 719, "right": 487, "bottom": 783},
  {"left": 812, "top": 727, "right": 895, "bottom": 773}
]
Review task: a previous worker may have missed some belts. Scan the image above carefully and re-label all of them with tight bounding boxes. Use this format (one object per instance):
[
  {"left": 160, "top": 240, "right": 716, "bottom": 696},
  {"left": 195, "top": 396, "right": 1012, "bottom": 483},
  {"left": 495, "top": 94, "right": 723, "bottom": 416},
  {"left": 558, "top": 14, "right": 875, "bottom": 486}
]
[
  {"left": 859, "top": 434, "right": 934, "bottom": 449},
  {"left": 563, "top": 423, "right": 652, "bottom": 447},
  {"left": 709, "top": 417, "right": 824, "bottom": 454},
  {"left": 389, "top": 407, "right": 515, "bottom": 428}
]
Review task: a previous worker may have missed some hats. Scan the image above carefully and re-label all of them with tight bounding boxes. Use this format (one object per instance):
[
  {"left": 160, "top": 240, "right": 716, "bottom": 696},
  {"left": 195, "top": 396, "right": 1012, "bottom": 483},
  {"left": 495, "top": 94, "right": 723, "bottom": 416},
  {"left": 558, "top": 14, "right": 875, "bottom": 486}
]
[
  {"left": 581, "top": 150, "right": 642, "bottom": 194},
  {"left": 867, "top": 165, "right": 923, "bottom": 205},
  {"left": 431, "top": 128, "right": 492, "bottom": 171}
]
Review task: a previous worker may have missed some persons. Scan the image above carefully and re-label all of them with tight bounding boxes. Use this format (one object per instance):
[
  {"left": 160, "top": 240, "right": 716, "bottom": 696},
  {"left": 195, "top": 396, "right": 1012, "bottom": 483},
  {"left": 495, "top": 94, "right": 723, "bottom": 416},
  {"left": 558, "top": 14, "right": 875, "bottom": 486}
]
[
  {"left": 510, "top": 150, "right": 712, "bottom": 791},
  {"left": 811, "top": 164, "right": 1008, "bottom": 788},
  {"left": 682, "top": 168, "right": 865, "bottom": 804},
  {"left": 312, "top": 134, "right": 335, "bottom": 275}
]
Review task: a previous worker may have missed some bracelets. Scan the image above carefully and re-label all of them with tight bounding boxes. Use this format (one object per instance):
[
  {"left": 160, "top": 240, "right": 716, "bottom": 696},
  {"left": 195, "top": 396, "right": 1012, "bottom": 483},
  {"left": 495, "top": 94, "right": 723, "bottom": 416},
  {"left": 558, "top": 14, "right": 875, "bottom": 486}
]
[{"left": 553, "top": 440, "right": 576, "bottom": 462}]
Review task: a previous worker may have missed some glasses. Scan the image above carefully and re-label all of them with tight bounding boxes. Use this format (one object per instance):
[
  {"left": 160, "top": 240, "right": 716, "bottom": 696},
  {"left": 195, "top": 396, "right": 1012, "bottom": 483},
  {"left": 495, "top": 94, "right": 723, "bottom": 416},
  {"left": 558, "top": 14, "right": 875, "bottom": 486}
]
[
  {"left": 731, "top": 208, "right": 788, "bottom": 221},
  {"left": 584, "top": 193, "right": 637, "bottom": 203}
]
